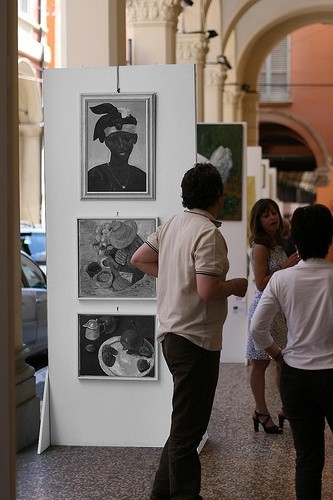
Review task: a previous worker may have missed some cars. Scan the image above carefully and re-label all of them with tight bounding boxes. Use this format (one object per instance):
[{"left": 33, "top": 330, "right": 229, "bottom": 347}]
[{"left": 20, "top": 220, "right": 48, "bottom": 365}]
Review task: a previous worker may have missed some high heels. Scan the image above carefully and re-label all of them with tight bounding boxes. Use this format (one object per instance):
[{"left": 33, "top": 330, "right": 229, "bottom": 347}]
[
  {"left": 278, "top": 408, "right": 288, "bottom": 428},
  {"left": 253, "top": 410, "right": 283, "bottom": 434}
]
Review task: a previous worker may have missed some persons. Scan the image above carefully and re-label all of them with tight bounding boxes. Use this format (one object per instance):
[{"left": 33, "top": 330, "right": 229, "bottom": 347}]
[
  {"left": 248, "top": 199, "right": 302, "bottom": 434},
  {"left": 250, "top": 203, "right": 333, "bottom": 500},
  {"left": 130, "top": 164, "right": 249, "bottom": 500}
]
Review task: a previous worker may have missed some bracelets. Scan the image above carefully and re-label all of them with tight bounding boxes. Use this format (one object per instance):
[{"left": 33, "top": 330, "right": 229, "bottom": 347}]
[{"left": 273, "top": 348, "right": 284, "bottom": 362}]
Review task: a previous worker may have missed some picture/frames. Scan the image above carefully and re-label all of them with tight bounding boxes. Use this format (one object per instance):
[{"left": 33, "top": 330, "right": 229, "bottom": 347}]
[
  {"left": 76, "top": 312, "right": 159, "bottom": 381},
  {"left": 80, "top": 92, "right": 156, "bottom": 201},
  {"left": 76, "top": 216, "right": 159, "bottom": 300},
  {"left": 196, "top": 124, "right": 242, "bottom": 220}
]
